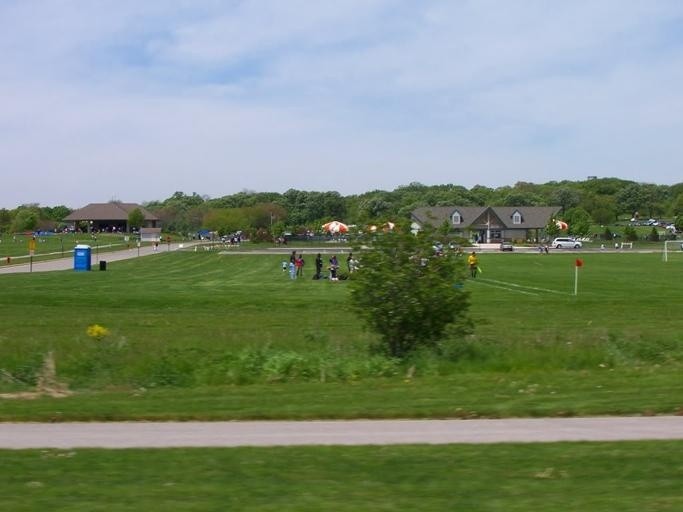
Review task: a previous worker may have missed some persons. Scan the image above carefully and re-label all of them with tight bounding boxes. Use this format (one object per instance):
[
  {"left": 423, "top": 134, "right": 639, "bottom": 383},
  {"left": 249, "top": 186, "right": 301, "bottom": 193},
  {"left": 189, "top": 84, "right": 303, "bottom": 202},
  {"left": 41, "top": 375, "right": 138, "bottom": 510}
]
[
  {"left": 615, "top": 242, "right": 620, "bottom": 249},
  {"left": 545, "top": 245, "right": 550, "bottom": 256},
  {"left": 220, "top": 233, "right": 241, "bottom": 245},
  {"left": 279, "top": 248, "right": 363, "bottom": 281},
  {"left": 600, "top": 243, "right": 606, "bottom": 250},
  {"left": 538, "top": 245, "right": 544, "bottom": 256},
  {"left": 468, "top": 251, "right": 478, "bottom": 278}
]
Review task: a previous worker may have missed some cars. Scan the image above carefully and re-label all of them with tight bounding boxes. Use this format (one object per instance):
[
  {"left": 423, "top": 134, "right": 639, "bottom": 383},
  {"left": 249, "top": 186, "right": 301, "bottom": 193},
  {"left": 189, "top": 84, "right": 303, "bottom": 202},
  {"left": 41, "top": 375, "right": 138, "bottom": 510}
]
[
  {"left": 500, "top": 241, "right": 512, "bottom": 252},
  {"left": 432, "top": 242, "right": 454, "bottom": 251},
  {"left": 630, "top": 218, "right": 681, "bottom": 233}
]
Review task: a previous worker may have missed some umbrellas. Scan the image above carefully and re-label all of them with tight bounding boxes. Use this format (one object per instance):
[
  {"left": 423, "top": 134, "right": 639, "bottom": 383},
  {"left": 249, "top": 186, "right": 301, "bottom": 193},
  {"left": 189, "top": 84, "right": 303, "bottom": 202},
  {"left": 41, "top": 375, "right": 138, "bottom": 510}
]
[
  {"left": 321, "top": 220, "right": 349, "bottom": 233},
  {"left": 553, "top": 218, "right": 568, "bottom": 230},
  {"left": 196, "top": 229, "right": 209, "bottom": 235}
]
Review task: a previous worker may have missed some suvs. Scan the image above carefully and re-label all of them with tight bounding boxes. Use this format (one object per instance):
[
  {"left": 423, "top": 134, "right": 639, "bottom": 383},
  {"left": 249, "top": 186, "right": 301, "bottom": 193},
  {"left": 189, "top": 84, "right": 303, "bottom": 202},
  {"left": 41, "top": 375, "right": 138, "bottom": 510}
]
[
  {"left": 553, "top": 237, "right": 582, "bottom": 249},
  {"left": 66, "top": 226, "right": 75, "bottom": 234}
]
